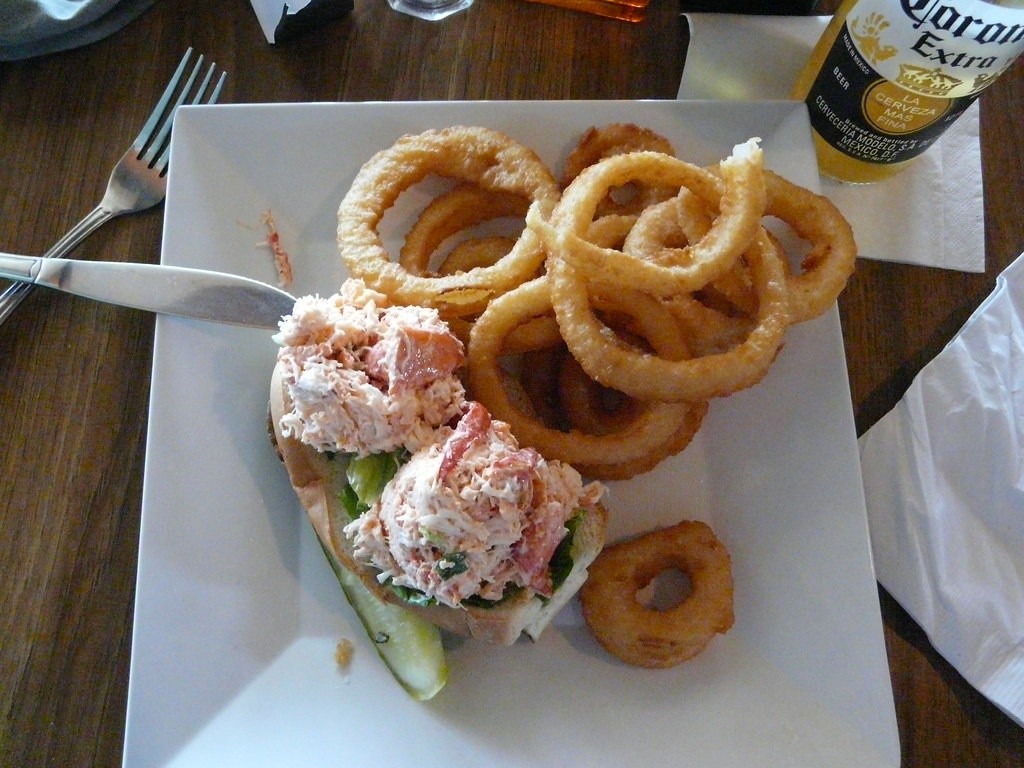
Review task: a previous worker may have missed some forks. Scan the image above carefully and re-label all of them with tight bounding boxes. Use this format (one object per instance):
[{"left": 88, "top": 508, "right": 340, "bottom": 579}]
[{"left": 0, "top": 47, "right": 226, "bottom": 323}]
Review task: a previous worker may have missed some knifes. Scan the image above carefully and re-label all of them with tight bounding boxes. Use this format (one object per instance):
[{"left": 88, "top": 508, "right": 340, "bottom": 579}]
[{"left": 0, "top": 252, "right": 295, "bottom": 332}]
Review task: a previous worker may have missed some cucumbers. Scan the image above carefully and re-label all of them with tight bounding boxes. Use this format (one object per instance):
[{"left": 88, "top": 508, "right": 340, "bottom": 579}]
[{"left": 316, "top": 547, "right": 449, "bottom": 704}]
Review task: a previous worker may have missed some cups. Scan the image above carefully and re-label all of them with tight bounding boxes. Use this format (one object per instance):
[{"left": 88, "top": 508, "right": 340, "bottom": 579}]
[{"left": 387, "top": 0, "right": 472, "bottom": 22}]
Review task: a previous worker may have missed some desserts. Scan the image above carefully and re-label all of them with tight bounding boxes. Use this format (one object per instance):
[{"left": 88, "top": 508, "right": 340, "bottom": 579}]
[{"left": 270, "top": 278, "right": 608, "bottom": 646}]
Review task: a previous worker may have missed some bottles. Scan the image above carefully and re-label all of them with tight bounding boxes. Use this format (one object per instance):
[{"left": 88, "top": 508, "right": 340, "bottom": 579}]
[{"left": 789, "top": 0, "right": 1024, "bottom": 184}]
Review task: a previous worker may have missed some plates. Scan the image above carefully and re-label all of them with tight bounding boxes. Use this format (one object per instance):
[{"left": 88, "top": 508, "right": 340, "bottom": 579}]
[{"left": 123, "top": 97, "right": 901, "bottom": 768}]
